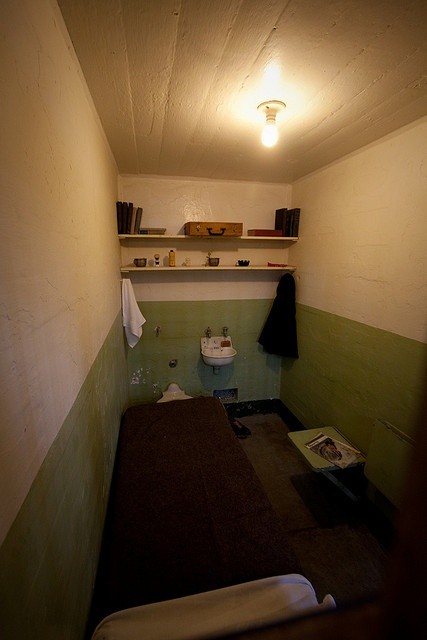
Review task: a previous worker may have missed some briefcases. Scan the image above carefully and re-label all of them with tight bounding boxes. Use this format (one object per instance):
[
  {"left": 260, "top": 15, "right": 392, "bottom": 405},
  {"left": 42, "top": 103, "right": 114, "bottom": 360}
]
[{"left": 183, "top": 222, "right": 243, "bottom": 237}]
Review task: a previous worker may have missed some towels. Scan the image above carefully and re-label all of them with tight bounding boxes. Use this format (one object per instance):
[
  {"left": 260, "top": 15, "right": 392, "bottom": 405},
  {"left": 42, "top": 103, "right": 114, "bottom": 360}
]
[{"left": 121, "top": 278, "right": 146, "bottom": 350}]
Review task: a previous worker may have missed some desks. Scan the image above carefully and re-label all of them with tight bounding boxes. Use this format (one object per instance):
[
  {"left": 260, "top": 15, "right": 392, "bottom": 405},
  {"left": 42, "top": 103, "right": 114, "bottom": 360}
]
[{"left": 286, "top": 426, "right": 370, "bottom": 502}]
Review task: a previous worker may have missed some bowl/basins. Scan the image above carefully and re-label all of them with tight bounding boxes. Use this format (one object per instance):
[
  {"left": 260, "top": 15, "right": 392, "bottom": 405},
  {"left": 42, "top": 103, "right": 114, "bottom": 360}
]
[
  {"left": 208, "top": 258, "right": 220, "bottom": 266},
  {"left": 133, "top": 258, "right": 148, "bottom": 267}
]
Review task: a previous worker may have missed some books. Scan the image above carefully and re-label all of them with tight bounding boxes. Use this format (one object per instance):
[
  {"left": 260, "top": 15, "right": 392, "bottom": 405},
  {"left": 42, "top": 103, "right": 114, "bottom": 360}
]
[
  {"left": 137, "top": 227, "right": 166, "bottom": 236},
  {"left": 116, "top": 201, "right": 142, "bottom": 235},
  {"left": 305, "top": 432, "right": 361, "bottom": 468},
  {"left": 274, "top": 207, "right": 301, "bottom": 237},
  {"left": 248, "top": 229, "right": 282, "bottom": 237}
]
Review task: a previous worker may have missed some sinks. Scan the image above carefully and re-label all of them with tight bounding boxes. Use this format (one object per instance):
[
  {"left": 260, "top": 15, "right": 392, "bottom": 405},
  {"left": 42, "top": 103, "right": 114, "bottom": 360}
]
[{"left": 200, "top": 337, "right": 237, "bottom": 366}]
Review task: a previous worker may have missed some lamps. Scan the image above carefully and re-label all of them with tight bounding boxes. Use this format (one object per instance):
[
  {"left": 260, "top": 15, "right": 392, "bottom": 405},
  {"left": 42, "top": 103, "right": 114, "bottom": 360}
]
[{"left": 255, "top": 101, "right": 290, "bottom": 150}]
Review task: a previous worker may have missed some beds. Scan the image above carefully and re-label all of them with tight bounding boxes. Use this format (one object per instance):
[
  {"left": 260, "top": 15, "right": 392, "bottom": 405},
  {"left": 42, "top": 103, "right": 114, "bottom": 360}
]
[{"left": 87, "top": 396, "right": 335, "bottom": 639}]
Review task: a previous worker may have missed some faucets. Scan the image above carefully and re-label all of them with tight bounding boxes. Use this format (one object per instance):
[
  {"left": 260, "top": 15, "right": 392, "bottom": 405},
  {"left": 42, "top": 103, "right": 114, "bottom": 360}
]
[
  {"left": 205, "top": 327, "right": 212, "bottom": 338},
  {"left": 223, "top": 327, "right": 228, "bottom": 337}
]
